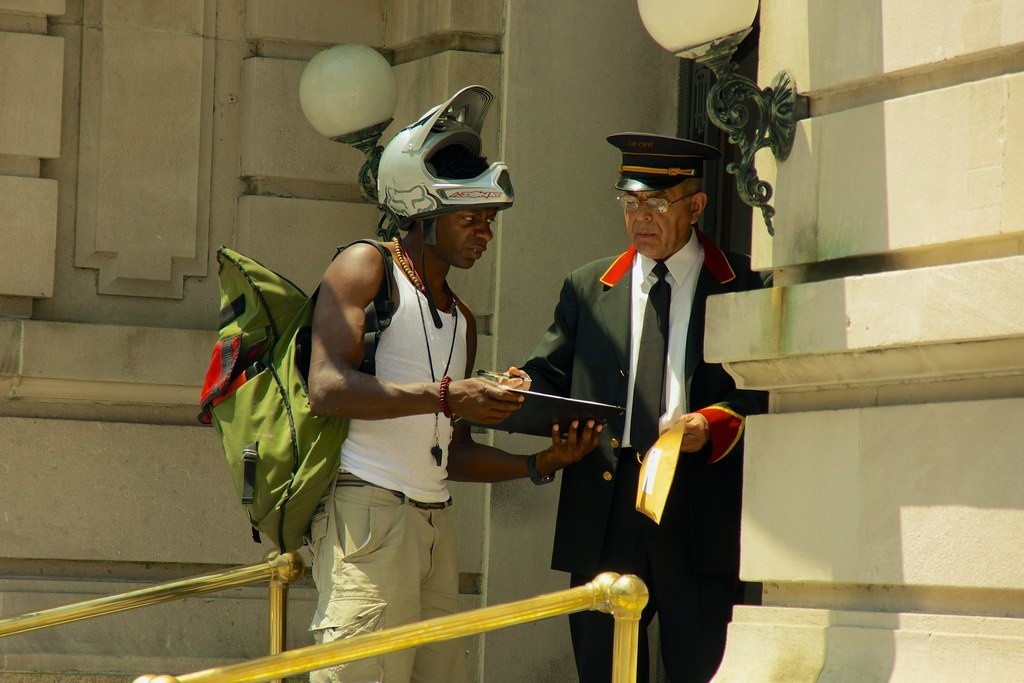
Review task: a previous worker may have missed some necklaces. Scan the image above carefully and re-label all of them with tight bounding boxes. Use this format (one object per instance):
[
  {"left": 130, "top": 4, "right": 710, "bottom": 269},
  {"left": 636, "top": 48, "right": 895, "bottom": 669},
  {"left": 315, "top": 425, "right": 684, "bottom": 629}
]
[{"left": 391, "top": 235, "right": 464, "bottom": 315}]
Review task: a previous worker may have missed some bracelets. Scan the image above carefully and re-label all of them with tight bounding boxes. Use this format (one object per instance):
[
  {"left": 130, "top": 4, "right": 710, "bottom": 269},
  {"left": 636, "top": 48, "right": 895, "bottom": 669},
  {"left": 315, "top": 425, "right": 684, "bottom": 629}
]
[{"left": 440, "top": 375, "right": 454, "bottom": 420}]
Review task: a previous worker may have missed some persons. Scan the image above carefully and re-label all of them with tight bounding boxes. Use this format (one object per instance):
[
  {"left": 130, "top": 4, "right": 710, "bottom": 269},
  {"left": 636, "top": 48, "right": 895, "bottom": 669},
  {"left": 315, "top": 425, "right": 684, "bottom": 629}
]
[
  {"left": 300, "top": 152, "right": 605, "bottom": 683},
  {"left": 491, "top": 131, "right": 771, "bottom": 682}
]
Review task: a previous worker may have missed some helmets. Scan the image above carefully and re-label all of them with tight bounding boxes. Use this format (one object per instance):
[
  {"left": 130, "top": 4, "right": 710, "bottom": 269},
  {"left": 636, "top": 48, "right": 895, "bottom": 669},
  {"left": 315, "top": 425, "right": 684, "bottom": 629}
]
[{"left": 377, "top": 85, "right": 512, "bottom": 229}]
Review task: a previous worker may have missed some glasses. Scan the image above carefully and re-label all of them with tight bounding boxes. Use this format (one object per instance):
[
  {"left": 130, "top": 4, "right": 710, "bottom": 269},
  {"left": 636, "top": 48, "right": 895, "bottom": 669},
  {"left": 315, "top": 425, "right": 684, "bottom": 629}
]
[{"left": 618, "top": 190, "right": 701, "bottom": 213}]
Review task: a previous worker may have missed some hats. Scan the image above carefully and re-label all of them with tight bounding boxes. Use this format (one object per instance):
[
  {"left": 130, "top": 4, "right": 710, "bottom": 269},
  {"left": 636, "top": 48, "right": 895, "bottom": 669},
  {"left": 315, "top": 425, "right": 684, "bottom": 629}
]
[{"left": 607, "top": 134, "right": 723, "bottom": 192}]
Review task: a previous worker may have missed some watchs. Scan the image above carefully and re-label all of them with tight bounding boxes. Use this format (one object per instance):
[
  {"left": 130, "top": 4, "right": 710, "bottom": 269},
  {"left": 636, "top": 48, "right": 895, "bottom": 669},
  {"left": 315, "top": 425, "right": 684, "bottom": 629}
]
[{"left": 527, "top": 453, "right": 557, "bottom": 485}]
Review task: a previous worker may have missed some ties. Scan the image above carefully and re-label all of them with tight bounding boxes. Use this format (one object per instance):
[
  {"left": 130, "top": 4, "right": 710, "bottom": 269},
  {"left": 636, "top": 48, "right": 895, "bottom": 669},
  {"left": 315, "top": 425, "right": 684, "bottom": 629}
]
[{"left": 630, "top": 263, "right": 673, "bottom": 457}]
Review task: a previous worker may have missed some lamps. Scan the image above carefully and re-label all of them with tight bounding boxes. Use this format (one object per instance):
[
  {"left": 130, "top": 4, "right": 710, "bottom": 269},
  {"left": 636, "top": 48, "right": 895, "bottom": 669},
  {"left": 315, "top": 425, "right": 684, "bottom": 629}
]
[
  {"left": 638, "top": 1, "right": 810, "bottom": 236},
  {"left": 300, "top": 44, "right": 399, "bottom": 204}
]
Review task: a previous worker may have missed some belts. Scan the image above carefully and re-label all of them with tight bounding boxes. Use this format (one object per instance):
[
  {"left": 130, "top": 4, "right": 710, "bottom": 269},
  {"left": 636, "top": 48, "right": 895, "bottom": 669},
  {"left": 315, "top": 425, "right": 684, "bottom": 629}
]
[{"left": 330, "top": 473, "right": 453, "bottom": 509}]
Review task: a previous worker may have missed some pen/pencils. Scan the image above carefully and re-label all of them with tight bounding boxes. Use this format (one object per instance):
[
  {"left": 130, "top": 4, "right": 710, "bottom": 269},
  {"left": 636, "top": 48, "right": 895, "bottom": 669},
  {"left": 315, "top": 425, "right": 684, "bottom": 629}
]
[{"left": 475, "top": 369, "right": 532, "bottom": 382}]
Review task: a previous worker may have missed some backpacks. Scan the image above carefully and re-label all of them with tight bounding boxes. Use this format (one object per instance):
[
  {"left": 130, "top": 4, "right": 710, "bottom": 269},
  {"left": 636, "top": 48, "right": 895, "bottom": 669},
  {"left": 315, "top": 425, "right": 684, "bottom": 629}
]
[{"left": 197, "top": 239, "right": 406, "bottom": 550}]
